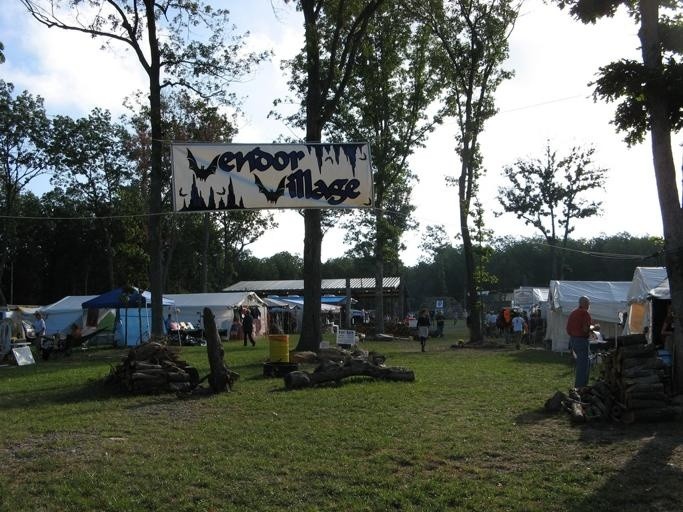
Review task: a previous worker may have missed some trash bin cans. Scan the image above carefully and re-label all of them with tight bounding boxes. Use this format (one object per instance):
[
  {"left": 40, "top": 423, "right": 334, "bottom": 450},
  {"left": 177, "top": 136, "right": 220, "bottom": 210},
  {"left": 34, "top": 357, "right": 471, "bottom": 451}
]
[{"left": 270, "top": 335, "right": 289, "bottom": 362}]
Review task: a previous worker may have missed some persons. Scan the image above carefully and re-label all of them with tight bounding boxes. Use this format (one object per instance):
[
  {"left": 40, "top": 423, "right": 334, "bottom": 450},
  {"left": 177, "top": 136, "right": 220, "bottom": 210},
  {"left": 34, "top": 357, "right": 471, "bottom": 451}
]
[
  {"left": 565, "top": 295, "right": 601, "bottom": 388},
  {"left": 661, "top": 304, "right": 674, "bottom": 374},
  {"left": 241, "top": 309, "right": 256, "bottom": 347},
  {"left": 165, "top": 313, "right": 174, "bottom": 333},
  {"left": 268, "top": 319, "right": 275, "bottom": 334},
  {"left": 66, "top": 322, "right": 80, "bottom": 343},
  {"left": 415, "top": 307, "right": 542, "bottom": 352},
  {"left": 32, "top": 312, "right": 47, "bottom": 351}
]
